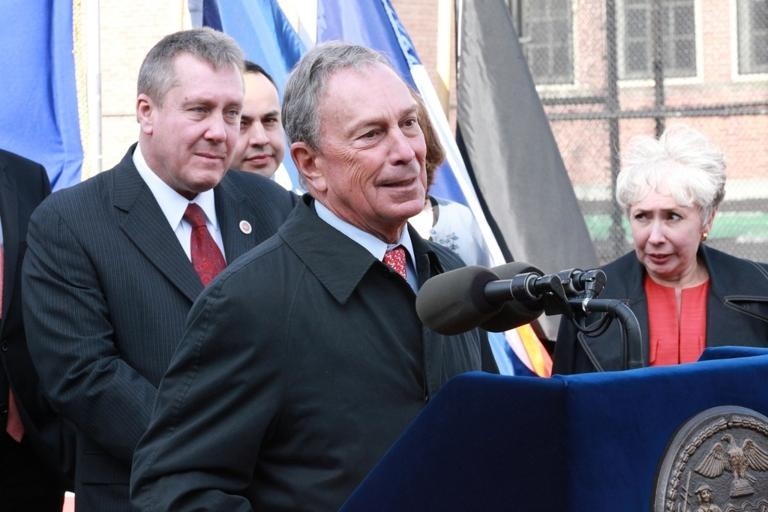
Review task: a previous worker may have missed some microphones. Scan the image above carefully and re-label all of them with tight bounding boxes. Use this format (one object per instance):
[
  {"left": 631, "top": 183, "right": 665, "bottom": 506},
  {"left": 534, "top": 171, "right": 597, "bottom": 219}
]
[
  {"left": 413, "top": 265, "right": 560, "bottom": 338},
  {"left": 479, "top": 259, "right": 606, "bottom": 335}
]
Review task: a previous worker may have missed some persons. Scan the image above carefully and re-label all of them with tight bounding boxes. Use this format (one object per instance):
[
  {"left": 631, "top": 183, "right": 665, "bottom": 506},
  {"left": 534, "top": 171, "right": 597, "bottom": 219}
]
[
  {"left": 551, "top": 122, "right": 768, "bottom": 377},
  {"left": 1, "top": 25, "right": 515, "bottom": 511}
]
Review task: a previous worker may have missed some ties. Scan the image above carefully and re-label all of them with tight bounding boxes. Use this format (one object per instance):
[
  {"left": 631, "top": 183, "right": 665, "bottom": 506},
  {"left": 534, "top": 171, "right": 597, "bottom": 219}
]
[
  {"left": 385, "top": 248, "right": 406, "bottom": 279},
  {"left": 182, "top": 203, "right": 227, "bottom": 287}
]
